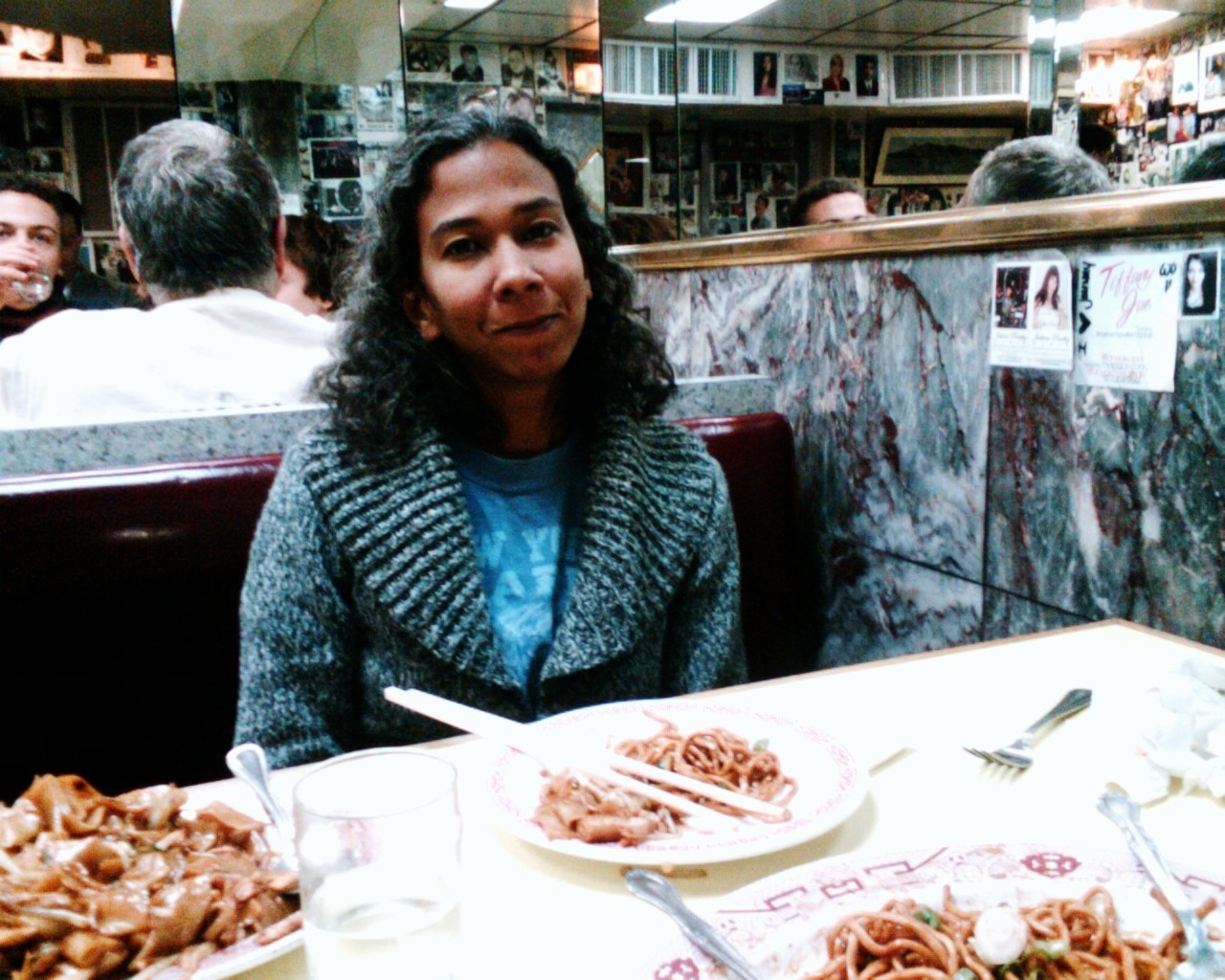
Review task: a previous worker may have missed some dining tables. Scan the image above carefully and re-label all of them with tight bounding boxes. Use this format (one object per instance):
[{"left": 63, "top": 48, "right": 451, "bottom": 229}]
[{"left": 0, "top": 619, "right": 1225, "bottom": 980}]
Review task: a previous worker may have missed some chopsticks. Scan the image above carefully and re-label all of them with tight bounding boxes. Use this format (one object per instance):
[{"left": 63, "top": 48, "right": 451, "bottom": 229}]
[{"left": 384, "top": 686, "right": 786, "bottom": 835}]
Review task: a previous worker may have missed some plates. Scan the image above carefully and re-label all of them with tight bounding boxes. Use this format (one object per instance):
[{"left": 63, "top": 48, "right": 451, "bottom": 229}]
[
  {"left": 25, "top": 785, "right": 381, "bottom": 980},
  {"left": 641, "top": 835, "right": 1225, "bottom": 980},
  {"left": 489, "top": 698, "right": 869, "bottom": 865}
]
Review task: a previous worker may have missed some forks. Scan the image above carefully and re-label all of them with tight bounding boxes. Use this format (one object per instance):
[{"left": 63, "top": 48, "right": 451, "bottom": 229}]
[{"left": 964, "top": 689, "right": 1091, "bottom": 769}]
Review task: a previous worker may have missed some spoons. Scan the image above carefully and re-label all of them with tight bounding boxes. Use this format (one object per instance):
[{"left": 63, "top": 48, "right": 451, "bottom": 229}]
[{"left": 1096, "top": 790, "right": 1225, "bottom": 980}]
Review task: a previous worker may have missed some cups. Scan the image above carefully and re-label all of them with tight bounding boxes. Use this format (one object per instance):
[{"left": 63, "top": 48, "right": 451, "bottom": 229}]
[
  {"left": 11, "top": 262, "right": 51, "bottom": 301},
  {"left": 293, "top": 751, "right": 461, "bottom": 980}
]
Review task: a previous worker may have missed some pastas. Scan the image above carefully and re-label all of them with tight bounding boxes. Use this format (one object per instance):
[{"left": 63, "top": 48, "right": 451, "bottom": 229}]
[
  {"left": 605, "top": 707, "right": 798, "bottom": 825},
  {"left": 795, "top": 884, "right": 1216, "bottom": 980}
]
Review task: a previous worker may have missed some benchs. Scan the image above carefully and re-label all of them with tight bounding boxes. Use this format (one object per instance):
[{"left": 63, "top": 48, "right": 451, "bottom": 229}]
[{"left": 0, "top": 374, "right": 808, "bottom": 811}]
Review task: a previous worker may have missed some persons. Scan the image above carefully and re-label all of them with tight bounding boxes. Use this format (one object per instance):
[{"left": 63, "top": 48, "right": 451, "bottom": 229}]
[
  {"left": 867, "top": 189, "right": 934, "bottom": 220},
  {"left": 750, "top": 196, "right": 773, "bottom": 232},
  {"left": 822, "top": 53, "right": 850, "bottom": 92},
  {"left": 451, "top": 43, "right": 485, "bottom": 81},
  {"left": 463, "top": 90, "right": 536, "bottom": 133},
  {"left": 753, "top": 52, "right": 777, "bottom": 97},
  {"left": 785, "top": 52, "right": 819, "bottom": 82},
  {"left": 952, "top": 136, "right": 1113, "bottom": 210},
  {"left": 0, "top": 177, "right": 153, "bottom": 349},
  {"left": 997, "top": 269, "right": 1028, "bottom": 327},
  {"left": 1184, "top": 253, "right": 1216, "bottom": 314},
  {"left": 536, "top": 49, "right": 568, "bottom": 94},
  {"left": 502, "top": 46, "right": 536, "bottom": 87},
  {"left": 1117, "top": 54, "right": 1225, "bottom": 177},
  {"left": 787, "top": 180, "right": 867, "bottom": 226},
  {"left": 710, "top": 161, "right": 739, "bottom": 200},
  {"left": 0, "top": 119, "right": 363, "bottom": 431},
  {"left": 272, "top": 214, "right": 358, "bottom": 331},
  {"left": 178, "top": 82, "right": 214, "bottom": 108},
  {"left": 856, "top": 54, "right": 879, "bottom": 100},
  {"left": 234, "top": 113, "right": 746, "bottom": 777},
  {"left": 306, "top": 83, "right": 341, "bottom": 110},
  {"left": 1173, "top": 141, "right": 1225, "bottom": 184},
  {"left": 1035, "top": 266, "right": 1065, "bottom": 329}
]
